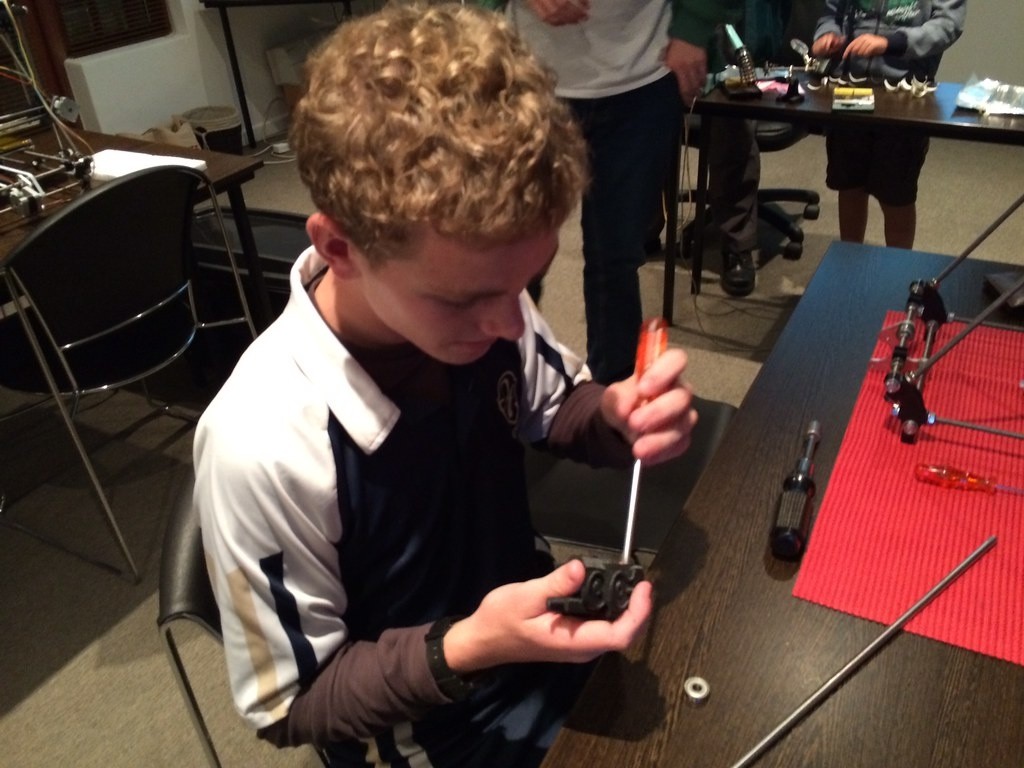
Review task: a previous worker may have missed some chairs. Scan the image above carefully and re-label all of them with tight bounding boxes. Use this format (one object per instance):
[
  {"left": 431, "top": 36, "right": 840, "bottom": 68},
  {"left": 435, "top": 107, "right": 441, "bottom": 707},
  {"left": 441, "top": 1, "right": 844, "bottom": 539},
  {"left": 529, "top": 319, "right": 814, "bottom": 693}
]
[
  {"left": 0, "top": 166, "right": 259, "bottom": 587},
  {"left": 537, "top": 395, "right": 738, "bottom": 565},
  {"left": 155, "top": 459, "right": 340, "bottom": 768}
]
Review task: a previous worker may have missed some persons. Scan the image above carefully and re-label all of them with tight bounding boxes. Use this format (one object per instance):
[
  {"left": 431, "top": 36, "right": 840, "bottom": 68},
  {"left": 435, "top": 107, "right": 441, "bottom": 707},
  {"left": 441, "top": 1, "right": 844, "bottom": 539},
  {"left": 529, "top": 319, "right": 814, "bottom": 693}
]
[
  {"left": 194, "top": 0, "right": 696, "bottom": 767},
  {"left": 472, "top": 2, "right": 710, "bottom": 385},
  {"left": 811, "top": 1, "right": 965, "bottom": 249},
  {"left": 647, "top": 0, "right": 824, "bottom": 295}
]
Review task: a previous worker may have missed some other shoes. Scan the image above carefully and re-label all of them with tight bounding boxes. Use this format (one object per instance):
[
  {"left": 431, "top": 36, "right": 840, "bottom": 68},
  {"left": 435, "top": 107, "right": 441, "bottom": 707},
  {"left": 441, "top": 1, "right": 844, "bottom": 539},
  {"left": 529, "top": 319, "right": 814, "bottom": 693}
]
[{"left": 721, "top": 246, "right": 755, "bottom": 295}]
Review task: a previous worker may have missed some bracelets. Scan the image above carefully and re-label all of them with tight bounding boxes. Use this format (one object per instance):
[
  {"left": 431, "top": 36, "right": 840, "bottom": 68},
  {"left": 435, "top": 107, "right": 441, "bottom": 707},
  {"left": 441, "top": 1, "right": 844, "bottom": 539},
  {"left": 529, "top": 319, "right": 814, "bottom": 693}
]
[{"left": 423, "top": 622, "right": 481, "bottom": 699}]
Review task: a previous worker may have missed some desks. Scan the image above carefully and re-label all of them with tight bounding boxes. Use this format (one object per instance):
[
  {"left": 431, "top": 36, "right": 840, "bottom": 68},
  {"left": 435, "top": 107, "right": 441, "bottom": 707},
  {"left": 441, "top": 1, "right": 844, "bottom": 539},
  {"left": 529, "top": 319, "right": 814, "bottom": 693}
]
[
  {"left": 538, "top": 77, "right": 1024, "bottom": 768},
  {"left": 0, "top": 0, "right": 387, "bottom": 339}
]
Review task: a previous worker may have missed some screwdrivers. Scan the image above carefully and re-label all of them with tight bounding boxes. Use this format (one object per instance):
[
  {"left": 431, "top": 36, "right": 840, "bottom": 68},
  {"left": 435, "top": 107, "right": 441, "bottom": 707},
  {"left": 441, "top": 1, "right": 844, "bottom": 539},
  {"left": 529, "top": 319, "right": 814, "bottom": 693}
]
[
  {"left": 616, "top": 316, "right": 669, "bottom": 567},
  {"left": 914, "top": 463, "right": 1023, "bottom": 497},
  {"left": 769, "top": 419, "right": 823, "bottom": 563}
]
